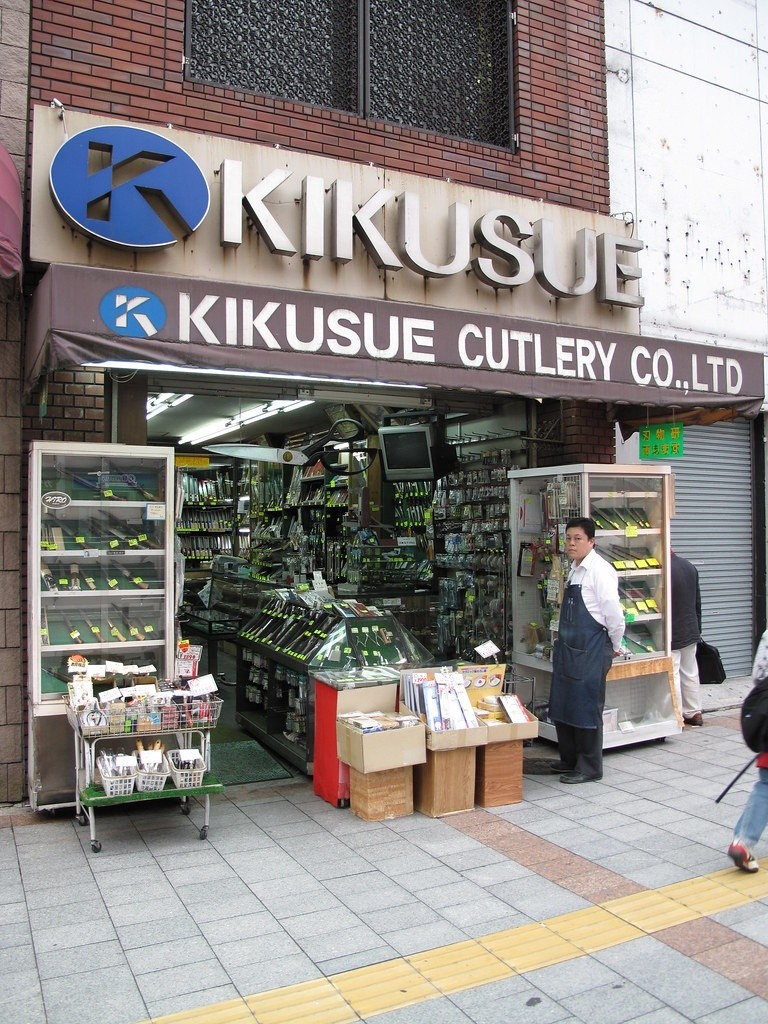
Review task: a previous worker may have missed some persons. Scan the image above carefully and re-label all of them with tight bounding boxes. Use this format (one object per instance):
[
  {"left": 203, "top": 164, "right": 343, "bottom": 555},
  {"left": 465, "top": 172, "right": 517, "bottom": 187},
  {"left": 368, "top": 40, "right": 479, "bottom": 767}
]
[
  {"left": 726, "top": 627, "right": 768, "bottom": 875},
  {"left": 549, "top": 517, "right": 625, "bottom": 784},
  {"left": 669, "top": 549, "right": 704, "bottom": 729}
]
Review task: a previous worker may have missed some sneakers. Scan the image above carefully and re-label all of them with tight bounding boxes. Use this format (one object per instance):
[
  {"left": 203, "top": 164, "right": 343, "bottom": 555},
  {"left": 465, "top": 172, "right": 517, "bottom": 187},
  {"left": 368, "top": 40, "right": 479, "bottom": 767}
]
[{"left": 729, "top": 839, "right": 758, "bottom": 872}]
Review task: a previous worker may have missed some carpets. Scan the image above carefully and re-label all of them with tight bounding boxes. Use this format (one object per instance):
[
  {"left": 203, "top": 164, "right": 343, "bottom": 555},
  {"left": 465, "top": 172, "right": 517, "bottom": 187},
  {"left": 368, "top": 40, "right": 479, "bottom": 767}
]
[{"left": 192, "top": 739, "right": 294, "bottom": 786}]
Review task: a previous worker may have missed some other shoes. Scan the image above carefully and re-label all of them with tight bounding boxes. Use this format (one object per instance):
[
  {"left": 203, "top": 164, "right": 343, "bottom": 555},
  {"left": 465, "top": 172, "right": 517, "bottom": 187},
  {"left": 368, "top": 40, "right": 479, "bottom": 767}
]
[
  {"left": 685, "top": 714, "right": 703, "bottom": 725},
  {"left": 560, "top": 772, "right": 602, "bottom": 783},
  {"left": 551, "top": 764, "right": 574, "bottom": 772}
]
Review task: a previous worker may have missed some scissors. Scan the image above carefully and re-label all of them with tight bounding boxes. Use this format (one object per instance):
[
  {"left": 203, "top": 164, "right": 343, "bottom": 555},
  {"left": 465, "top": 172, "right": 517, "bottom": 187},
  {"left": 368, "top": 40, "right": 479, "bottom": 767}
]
[{"left": 201, "top": 418, "right": 379, "bottom": 476}]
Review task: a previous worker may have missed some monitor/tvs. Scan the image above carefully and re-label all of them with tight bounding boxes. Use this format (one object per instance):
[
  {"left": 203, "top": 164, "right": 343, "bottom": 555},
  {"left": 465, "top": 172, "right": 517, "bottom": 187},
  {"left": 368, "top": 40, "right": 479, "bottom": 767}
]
[{"left": 378, "top": 423, "right": 436, "bottom": 480}]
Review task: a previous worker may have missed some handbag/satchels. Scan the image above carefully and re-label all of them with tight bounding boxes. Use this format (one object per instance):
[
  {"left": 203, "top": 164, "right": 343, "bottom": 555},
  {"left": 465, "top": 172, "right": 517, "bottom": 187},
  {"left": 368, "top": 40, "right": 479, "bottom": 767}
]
[{"left": 741, "top": 677, "right": 768, "bottom": 755}]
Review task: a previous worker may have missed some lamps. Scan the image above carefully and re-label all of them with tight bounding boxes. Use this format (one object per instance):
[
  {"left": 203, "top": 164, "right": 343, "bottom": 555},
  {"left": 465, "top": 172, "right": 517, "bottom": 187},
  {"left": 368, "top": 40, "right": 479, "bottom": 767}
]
[{"left": 146, "top": 393, "right": 315, "bottom": 445}]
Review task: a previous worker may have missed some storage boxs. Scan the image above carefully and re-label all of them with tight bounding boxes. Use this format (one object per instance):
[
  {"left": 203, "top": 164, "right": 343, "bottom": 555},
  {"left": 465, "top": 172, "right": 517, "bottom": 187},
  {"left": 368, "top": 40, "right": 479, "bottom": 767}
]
[
  {"left": 97, "top": 754, "right": 139, "bottom": 797},
  {"left": 62, "top": 690, "right": 225, "bottom": 739},
  {"left": 61, "top": 675, "right": 180, "bottom": 736},
  {"left": 131, "top": 750, "right": 172, "bottom": 792},
  {"left": 603, "top": 705, "right": 619, "bottom": 733},
  {"left": 167, "top": 748, "right": 207, "bottom": 789},
  {"left": 336, "top": 683, "right": 428, "bottom": 775},
  {"left": 398, "top": 666, "right": 488, "bottom": 751},
  {"left": 456, "top": 663, "right": 539, "bottom": 743}
]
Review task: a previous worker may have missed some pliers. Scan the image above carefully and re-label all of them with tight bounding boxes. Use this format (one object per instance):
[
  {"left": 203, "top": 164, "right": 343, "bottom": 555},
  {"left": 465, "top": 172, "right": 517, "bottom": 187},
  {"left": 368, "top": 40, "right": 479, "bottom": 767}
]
[{"left": 355, "top": 631, "right": 386, "bottom": 648}]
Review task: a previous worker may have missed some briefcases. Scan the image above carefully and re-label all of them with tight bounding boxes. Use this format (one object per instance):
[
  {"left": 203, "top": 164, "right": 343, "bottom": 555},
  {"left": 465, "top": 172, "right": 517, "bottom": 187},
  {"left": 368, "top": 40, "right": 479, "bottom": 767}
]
[{"left": 697, "top": 637, "right": 726, "bottom": 684}]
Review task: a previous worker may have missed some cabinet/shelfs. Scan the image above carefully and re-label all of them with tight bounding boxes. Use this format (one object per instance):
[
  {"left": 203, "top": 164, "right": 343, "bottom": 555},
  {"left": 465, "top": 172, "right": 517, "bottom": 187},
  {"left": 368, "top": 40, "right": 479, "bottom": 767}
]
[
  {"left": 507, "top": 463, "right": 672, "bottom": 665},
  {"left": 27, "top": 441, "right": 175, "bottom": 706},
  {"left": 74, "top": 730, "right": 226, "bottom": 854},
  {"left": 176, "top": 426, "right": 528, "bottom": 776}
]
